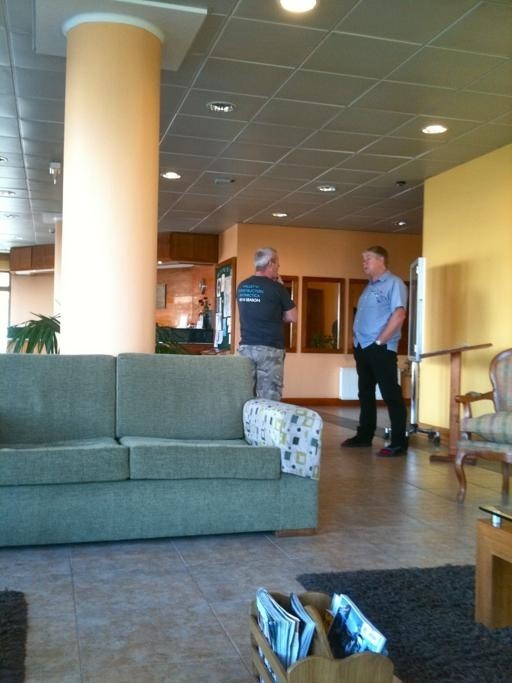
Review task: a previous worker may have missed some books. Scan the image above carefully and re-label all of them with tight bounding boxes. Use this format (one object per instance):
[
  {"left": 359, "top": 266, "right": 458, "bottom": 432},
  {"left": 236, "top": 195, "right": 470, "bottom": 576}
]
[
  {"left": 254, "top": 585, "right": 299, "bottom": 682},
  {"left": 288, "top": 591, "right": 316, "bottom": 661},
  {"left": 327, "top": 594, "right": 387, "bottom": 661}
]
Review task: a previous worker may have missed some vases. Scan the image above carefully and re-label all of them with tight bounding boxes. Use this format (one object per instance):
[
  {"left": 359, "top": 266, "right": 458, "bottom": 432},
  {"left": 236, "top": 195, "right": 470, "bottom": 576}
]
[{"left": 201, "top": 309, "right": 211, "bottom": 329}]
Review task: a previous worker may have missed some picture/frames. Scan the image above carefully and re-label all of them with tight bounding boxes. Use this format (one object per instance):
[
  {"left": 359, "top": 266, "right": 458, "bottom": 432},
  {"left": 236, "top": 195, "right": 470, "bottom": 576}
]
[{"left": 214, "top": 257, "right": 237, "bottom": 353}]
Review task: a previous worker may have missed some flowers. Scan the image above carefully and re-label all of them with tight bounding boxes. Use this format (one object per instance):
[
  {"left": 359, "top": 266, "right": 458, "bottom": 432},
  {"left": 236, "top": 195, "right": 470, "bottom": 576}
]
[{"left": 199, "top": 296, "right": 212, "bottom": 328}]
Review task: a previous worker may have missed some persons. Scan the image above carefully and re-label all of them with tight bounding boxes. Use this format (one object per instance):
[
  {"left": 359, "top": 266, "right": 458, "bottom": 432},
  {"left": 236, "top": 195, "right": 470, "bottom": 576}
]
[
  {"left": 339, "top": 244, "right": 408, "bottom": 456},
  {"left": 236, "top": 244, "right": 298, "bottom": 402}
]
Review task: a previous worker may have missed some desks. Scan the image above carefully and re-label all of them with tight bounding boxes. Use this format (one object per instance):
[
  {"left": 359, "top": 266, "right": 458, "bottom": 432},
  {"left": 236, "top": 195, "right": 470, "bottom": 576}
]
[{"left": 474, "top": 507, "right": 510, "bottom": 631}]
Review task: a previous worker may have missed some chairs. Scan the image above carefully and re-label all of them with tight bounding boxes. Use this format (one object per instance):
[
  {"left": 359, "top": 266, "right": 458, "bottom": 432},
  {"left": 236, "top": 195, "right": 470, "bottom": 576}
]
[{"left": 453, "top": 347, "right": 512, "bottom": 504}]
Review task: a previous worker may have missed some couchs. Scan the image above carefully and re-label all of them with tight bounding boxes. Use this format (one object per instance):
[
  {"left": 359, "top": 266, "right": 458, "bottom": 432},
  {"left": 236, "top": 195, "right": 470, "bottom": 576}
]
[{"left": 0, "top": 352, "right": 325, "bottom": 547}]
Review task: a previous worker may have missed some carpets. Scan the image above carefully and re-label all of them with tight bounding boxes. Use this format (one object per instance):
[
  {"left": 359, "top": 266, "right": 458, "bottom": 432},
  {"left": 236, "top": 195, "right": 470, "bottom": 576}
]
[
  {"left": 1, "top": 589, "right": 26, "bottom": 682},
  {"left": 294, "top": 564, "right": 511, "bottom": 682}
]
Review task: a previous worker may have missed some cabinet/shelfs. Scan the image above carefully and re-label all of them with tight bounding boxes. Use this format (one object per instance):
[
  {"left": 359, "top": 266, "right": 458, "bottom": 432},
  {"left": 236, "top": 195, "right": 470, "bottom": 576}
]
[{"left": 249, "top": 589, "right": 394, "bottom": 682}]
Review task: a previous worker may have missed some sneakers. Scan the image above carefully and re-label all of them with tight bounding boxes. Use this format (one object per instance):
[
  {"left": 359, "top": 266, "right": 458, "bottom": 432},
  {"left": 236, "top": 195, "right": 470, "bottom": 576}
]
[
  {"left": 340, "top": 437, "right": 373, "bottom": 448},
  {"left": 375, "top": 445, "right": 407, "bottom": 457}
]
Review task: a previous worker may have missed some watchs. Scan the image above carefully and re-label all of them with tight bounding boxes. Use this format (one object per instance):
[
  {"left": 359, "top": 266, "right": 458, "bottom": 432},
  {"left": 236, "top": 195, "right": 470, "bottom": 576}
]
[{"left": 375, "top": 340, "right": 385, "bottom": 345}]
